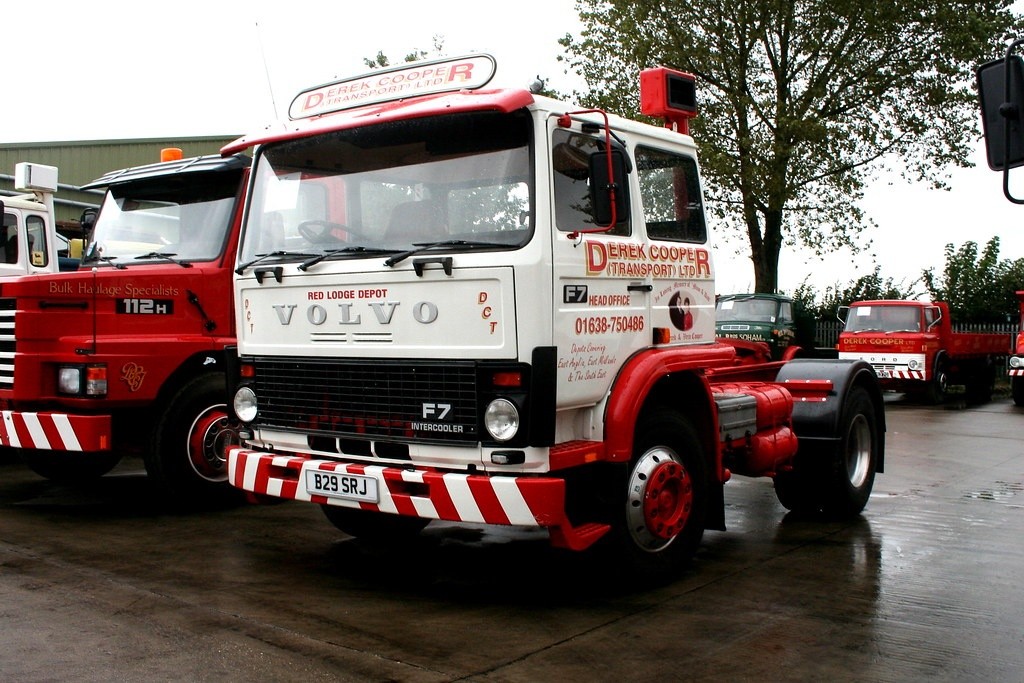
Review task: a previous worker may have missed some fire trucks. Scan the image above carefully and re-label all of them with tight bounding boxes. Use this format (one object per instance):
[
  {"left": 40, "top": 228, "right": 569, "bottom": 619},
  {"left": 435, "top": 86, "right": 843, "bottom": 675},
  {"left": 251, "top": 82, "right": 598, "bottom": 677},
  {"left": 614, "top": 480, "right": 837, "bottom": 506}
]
[
  {"left": 218, "top": 51, "right": 891, "bottom": 566},
  {"left": 1, "top": 147, "right": 350, "bottom": 508}
]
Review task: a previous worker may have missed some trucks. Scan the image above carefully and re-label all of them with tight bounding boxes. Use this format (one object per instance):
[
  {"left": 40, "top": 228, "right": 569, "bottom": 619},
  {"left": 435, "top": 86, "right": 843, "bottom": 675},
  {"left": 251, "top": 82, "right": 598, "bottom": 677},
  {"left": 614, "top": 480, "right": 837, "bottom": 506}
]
[
  {"left": 835, "top": 292, "right": 1017, "bottom": 401},
  {"left": 713, "top": 294, "right": 838, "bottom": 362}
]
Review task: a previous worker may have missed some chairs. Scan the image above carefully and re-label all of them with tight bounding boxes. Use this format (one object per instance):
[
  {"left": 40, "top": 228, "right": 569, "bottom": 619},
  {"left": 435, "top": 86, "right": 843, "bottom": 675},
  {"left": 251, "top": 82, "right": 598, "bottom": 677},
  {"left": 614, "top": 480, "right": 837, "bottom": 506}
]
[
  {"left": 5, "top": 233, "right": 35, "bottom": 264},
  {"left": 383, "top": 202, "right": 450, "bottom": 246}
]
